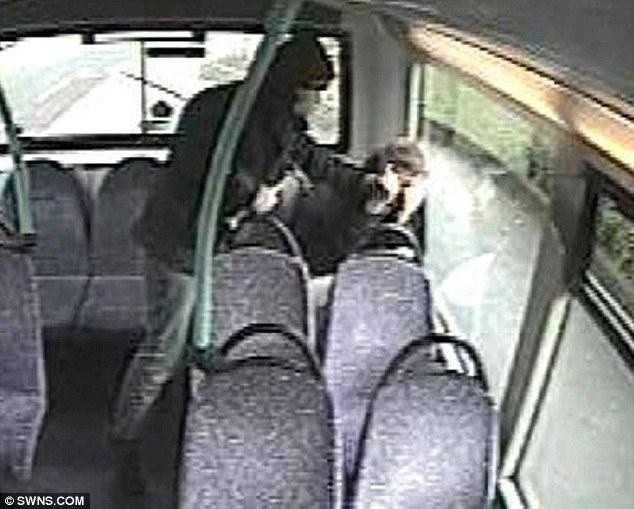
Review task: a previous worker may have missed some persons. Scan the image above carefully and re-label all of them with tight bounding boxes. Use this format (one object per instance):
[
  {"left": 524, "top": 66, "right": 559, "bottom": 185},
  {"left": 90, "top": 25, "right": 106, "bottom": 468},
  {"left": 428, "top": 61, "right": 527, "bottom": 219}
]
[
  {"left": 288, "top": 134, "right": 428, "bottom": 279},
  {"left": 107, "top": 29, "right": 399, "bottom": 498}
]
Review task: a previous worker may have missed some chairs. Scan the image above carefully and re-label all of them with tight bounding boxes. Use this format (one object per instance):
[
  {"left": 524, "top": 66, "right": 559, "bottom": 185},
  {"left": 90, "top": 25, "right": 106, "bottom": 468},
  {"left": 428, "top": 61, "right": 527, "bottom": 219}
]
[
  {"left": 208, "top": 211, "right": 316, "bottom": 362},
  {"left": 344, "top": 332, "right": 500, "bottom": 509},
  {"left": 175, "top": 321, "right": 344, "bottom": 509},
  {"left": 3, "top": 157, "right": 91, "bottom": 345},
  {"left": 75, "top": 157, "right": 166, "bottom": 344},
  {"left": 324, "top": 222, "right": 433, "bottom": 478},
  {"left": 0, "top": 249, "right": 50, "bottom": 482}
]
